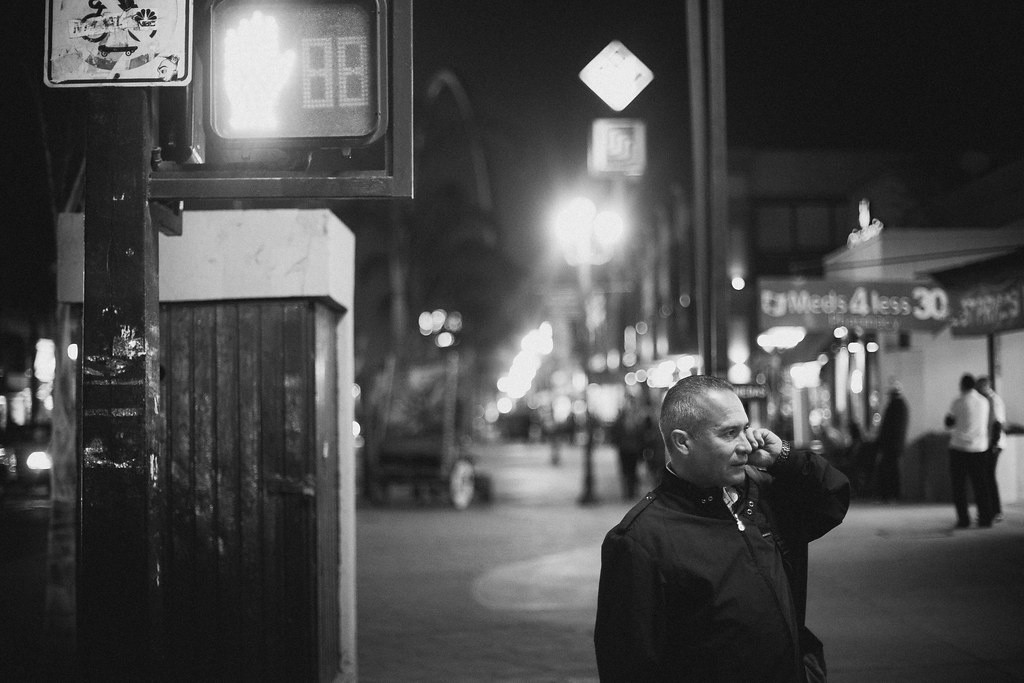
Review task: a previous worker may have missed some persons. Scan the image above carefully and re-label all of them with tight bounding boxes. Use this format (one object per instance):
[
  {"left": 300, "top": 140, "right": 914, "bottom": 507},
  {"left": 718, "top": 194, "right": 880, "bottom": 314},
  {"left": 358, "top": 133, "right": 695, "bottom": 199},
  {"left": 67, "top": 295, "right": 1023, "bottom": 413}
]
[
  {"left": 593, "top": 375, "right": 853, "bottom": 683},
  {"left": 577, "top": 386, "right": 667, "bottom": 503},
  {"left": 944, "top": 373, "right": 991, "bottom": 533},
  {"left": 869, "top": 386, "right": 910, "bottom": 506},
  {"left": 974, "top": 376, "right": 1006, "bottom": 525}
]
